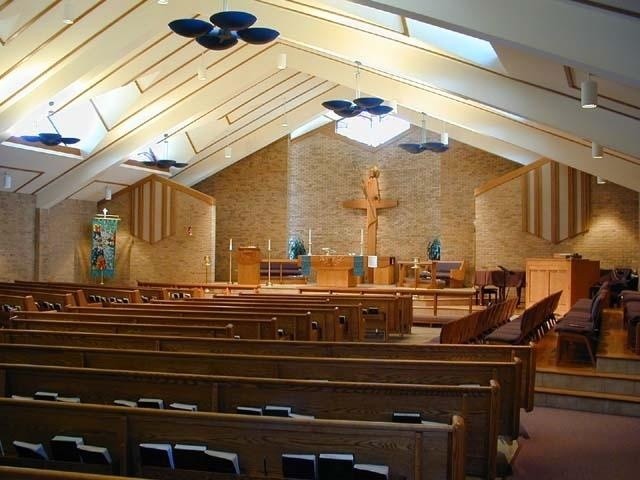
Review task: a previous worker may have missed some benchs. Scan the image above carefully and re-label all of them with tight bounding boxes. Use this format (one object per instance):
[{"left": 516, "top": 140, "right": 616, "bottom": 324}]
[
  {"left": 259, "top": 261, "right": 301, "bottom": 283},
  {"left": 421, "top": 261, "right": 466, "bottom": 288}
]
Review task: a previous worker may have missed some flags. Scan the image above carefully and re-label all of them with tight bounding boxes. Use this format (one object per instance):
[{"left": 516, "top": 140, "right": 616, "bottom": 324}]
[{"left": 87, "top": 217, "right": 120, "bottom": 280}]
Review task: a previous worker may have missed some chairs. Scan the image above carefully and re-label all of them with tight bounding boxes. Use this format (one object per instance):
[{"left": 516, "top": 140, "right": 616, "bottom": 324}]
[{"left": 425, "top": 268, "right": 639, "bottom": 369}]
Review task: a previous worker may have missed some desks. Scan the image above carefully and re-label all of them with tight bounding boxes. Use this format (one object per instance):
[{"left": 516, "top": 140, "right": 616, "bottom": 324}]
[
  {"left": 396, "top": 260, "right": 439, "bottom": 289},
  {"left": 524, "top": 256, "right": 600, "bottom": 321},
  {"left": 297, "top": 254, "right": 364, "bottom": 288}
]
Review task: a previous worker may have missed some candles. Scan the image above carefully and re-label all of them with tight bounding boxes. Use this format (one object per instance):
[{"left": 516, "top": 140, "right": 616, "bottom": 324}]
[
  {"left": 229, "top": 239, "right": 232, "bottom": 250},
  {"left": 360, "top": 229, "right": 363, "bottom": 242},
  {"left": 268, "top": 240, "right": 271, "bottom": 250},
  {"left": 308, "top": 230, "right": 312, "bottom": 244}
]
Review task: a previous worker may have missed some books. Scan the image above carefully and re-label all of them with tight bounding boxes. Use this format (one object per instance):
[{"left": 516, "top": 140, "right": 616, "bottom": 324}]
[
  {"left": 282, "top": 453, "right": 390, "bottom": 478},
  {"left": 139, "top": 444, "right": 240, "bottom": 476},
  {"left": 0, "top": 290, "right": 193, "bottom": 314},
  {"left": 113, "top": 398, "right": 197, "bottom": 412},
  {"left": 11, "top": 435, "right": 114, "bottom": 463},
  {"left": 392, "top": 411, "right": 448, "bottom": 426},
  {"left": 277, "top": 306, "right": 379, "bottom": 337},
  {"left": 237, "top": 406, "right": 315, "bottom": 420},
  {"left": 12, "top": 391, "right": 80, "bottom": 403}
]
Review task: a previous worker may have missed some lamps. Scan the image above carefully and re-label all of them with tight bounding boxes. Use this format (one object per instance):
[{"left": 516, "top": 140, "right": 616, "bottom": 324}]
[
  {"left": 2, "top": 170, "right": 12, "bottom": 190},
  {"left": 60, "top": 0, "right": 74, "bottom": 25},
  {"left": 167, "top": 1, "right": 287, "bottom": 81},
  {"left": 103, "top": 184, "right": 113, "bottom": 202},
  {"left": 21, "top": 101, "right": 81, "bottom": 147},
  {"left": 580, "top": 72, "right": 606, "bottom": 159},
  {"left": 320, "top": 60, "right": 450, "bottom": 156},
  {"left": 140, "top": 132, "right": 233, "bottom": 172},
  {"left": 281, "top": 115, "right": 288, "bottom": 127}
]
